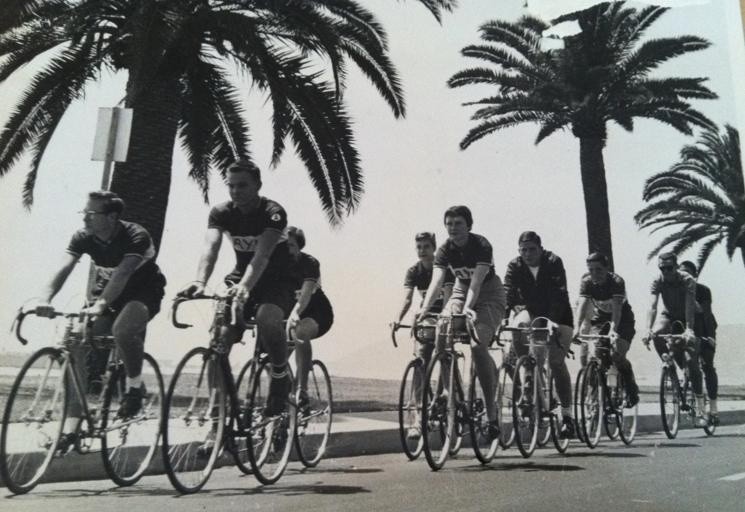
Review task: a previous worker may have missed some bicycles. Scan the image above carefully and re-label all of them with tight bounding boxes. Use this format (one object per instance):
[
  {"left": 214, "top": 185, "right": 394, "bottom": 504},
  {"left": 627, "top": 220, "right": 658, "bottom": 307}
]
[
  {"left": 160, "top": 284, "right": 299, "bottom": 493},
  {"left": 0, "top": 295, "right": 166, "bottom": 495},
  {"left": 231, "top": 308, "right": 334, "bottom": 475}
]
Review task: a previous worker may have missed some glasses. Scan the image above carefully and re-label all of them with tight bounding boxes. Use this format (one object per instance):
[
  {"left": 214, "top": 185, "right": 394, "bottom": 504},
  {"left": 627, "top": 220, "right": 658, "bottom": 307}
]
[
  {"left": 658, "top": 266, "right": 675, "bottom": 272},
  {"left": 84, "top": 208, "right": 109, "bottom": 218}
]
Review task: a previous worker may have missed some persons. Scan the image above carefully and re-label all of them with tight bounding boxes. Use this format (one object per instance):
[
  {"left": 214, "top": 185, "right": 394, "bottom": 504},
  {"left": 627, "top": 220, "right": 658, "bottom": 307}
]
[
  {"left": 171, "top": 159, "right": 304, "bottom": 455},
  {"left": 646, "top": 253, "right": 707, "bottom": 427},
  {"left": 569, "top": 252, "right": 641, "bottom": 407},
  {"left": 37, "top": 190, "right": 167, "bottom": 455},
  {"left": 413, "top": 204, "right": 506, "bottom": 439},
  {"left": 270, "top": 226, "right": 334, "bottom": 451},
  {"left": 679, "top": 261, "right": 719, "bottom": 417},
  {"left": 578, "top": 273, "right": 602, "bottom": 368},
  {"left": 505, "top": 232, "right": 575, "bottom": 435},
  {"left": 389, "top": 231, "right": 456, "bottom": 439}
]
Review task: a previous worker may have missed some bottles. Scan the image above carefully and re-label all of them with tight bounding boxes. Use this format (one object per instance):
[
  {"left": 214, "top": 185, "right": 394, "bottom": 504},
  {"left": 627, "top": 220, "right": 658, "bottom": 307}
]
[
  {"left": 662, "top": 353, "right": 673, "bottom": 367},
  {"left": 605, "top": 365, "right": 617, "bottom": 388}
]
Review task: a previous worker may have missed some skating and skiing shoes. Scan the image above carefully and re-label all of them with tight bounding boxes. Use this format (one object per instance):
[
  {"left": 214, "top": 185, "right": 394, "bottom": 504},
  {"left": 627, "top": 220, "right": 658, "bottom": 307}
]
[{"left": 477, "top": 421, "right": 501, "bottom": 447}]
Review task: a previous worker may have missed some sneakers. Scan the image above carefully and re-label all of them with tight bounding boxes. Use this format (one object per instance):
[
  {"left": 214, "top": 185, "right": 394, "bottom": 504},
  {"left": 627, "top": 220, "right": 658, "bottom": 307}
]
[
  {"left": 560, "top": 420, "right": 576, "bottom": 439},
  {"left": 405, "top": 414, "right": 424, "bottom": 440},
  {"left": 695, "top": 395, "right": 719, "bottom": 423},
  {"left": 116, "top": 383, "right": 147, "bottom": 420},
  {"left": 261, "top": 376, "right": 296, "bottom": 416},
  {"left": 625, "top": 396, "right": 640, "bottom": 408},
  {"left": 44, "top": 434, "right": 78, "bottom": 450},
  {"left": 194, "top": 422, "right": 230, "bottom": 461}
]
